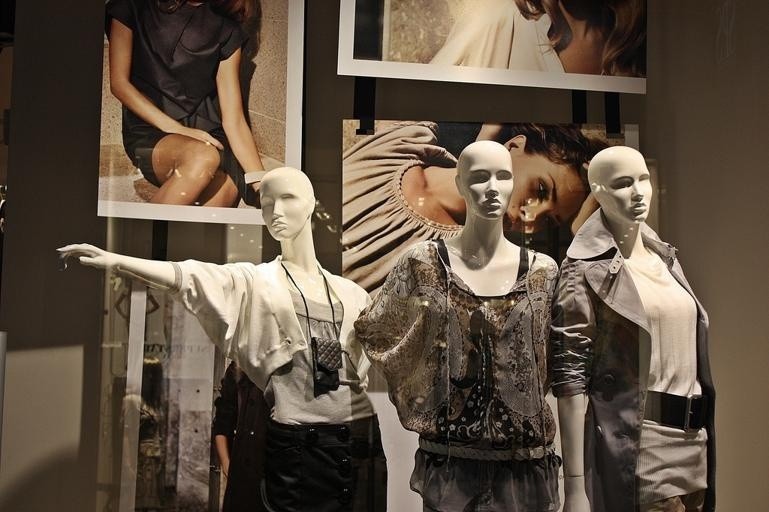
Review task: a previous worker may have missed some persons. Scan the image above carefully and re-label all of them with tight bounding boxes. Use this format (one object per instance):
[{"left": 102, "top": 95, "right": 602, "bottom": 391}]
[
  {"left": 427, "top": 1, "right": 648, "bottom": 78},
  {"left": 56, "top": 164, "right": 388, "bottom": 511},
  {"left": 103, "top": 0, "right": 264, "bottom": 206},
  {"left": 546, "top": 146, "right": 719, "bottom": 512},
  {"left": 213, "top": 360, "right": 238, "bottom": 483},
  {"left": 117, "top": 353, "right": 169, "bottom": 512},
  {"left": 353, "top": 138, "right": 559, "bottom": 511},
  {"left": 343, "top": 120, "right": 607, "bottom": 295}
]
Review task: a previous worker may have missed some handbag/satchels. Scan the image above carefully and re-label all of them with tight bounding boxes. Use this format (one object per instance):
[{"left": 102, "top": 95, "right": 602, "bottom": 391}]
[{"left": 309, "top": 335, "right": 343, "bottom": 391}]
